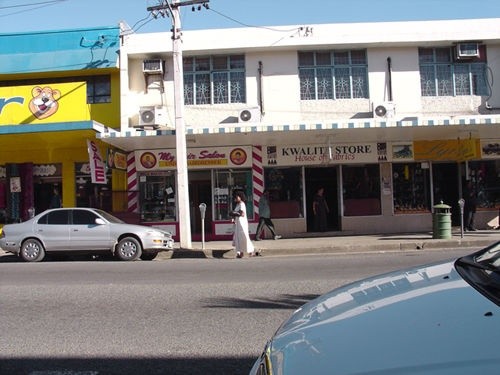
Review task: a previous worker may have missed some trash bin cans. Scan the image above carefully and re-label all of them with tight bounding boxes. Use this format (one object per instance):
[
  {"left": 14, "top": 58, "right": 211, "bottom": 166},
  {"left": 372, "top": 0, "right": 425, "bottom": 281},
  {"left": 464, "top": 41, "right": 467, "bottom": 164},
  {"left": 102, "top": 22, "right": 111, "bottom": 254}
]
[{"left": 432, "top": 200, "right": 453, "bottom": 239}]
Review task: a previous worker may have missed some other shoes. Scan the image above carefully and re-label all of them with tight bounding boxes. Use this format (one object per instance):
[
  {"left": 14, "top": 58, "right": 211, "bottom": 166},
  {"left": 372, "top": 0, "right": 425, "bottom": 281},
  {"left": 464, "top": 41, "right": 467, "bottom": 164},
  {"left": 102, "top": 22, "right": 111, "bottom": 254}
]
[
  {"left": 237, "top": 254, "right": 245, "bottom": 258},
  {"left": 470, "top": 227, "right": 476, "bottom": 231},
  {"left": 249, "top": 251, "right": 254, "bottom": 256},
  {"left": 465, "top": 228, "right": 469, "bottom": 232}
]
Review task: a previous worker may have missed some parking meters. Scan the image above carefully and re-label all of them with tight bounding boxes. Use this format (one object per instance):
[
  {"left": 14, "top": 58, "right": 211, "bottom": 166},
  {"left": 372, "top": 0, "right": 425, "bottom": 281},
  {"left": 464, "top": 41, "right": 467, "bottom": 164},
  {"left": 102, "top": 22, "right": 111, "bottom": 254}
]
[
  {"left": 198, "top": 203, "right": 207, "bottom": 248},
  {"left": 459, "top": 198, "right": 465, "bottom": 238}
]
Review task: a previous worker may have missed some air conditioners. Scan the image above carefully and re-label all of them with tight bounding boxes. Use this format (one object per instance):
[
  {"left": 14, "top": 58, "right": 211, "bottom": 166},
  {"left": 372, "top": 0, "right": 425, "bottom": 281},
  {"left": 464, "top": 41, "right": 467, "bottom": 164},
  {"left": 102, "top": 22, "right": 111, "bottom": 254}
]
[
  {"left": 457, "top": 42, "right": 479, "bottom": 59},
  {"left": 372, "top": 103, "right": 394, "bottom": 118},
  {"left": 138, "top": 108, "right": 164, "bottom": 126},
  {"left": 142, "top": 58, "right": 163, "bottom": 74},
  {"left": 238, "top": 110, "right": 260, "bottom": 123}
]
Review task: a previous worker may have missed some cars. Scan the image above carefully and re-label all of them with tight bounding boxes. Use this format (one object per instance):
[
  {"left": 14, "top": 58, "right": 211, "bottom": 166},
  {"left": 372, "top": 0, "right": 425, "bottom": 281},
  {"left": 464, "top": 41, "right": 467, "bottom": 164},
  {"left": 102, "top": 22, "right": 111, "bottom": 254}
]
[
  {"left": 0, "top": 208, "right": 174, "bottom": 261},
  {"left": 248, "top": 240, "right": 500, "bottom": 375}
]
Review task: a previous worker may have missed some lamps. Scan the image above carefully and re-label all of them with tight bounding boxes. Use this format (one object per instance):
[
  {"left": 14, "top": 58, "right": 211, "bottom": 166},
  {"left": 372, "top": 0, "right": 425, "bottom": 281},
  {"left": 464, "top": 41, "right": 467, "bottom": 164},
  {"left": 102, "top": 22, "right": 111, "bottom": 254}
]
[
  {"left": 457, "top": 199, "right": 466, "bottom": 209},
  {"left": 199, "top": 203, "right": 207, "bottom": 214}
]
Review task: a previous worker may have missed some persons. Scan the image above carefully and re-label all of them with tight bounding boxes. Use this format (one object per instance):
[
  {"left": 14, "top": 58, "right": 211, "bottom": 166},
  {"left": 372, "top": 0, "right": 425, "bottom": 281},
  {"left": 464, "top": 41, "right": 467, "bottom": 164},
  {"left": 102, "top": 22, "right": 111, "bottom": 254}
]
[
  {"left": 312, "top": 186, "right": 329, "bottom": 232},
  {"left": 464, "top": 184, "right": 479, "bottom": 231},
  {"left": 229, "top": 191, "right": 254, "bottom": 258},
  {"left": 254, "top": 190, "right": 282, "bottom": 240}
]
